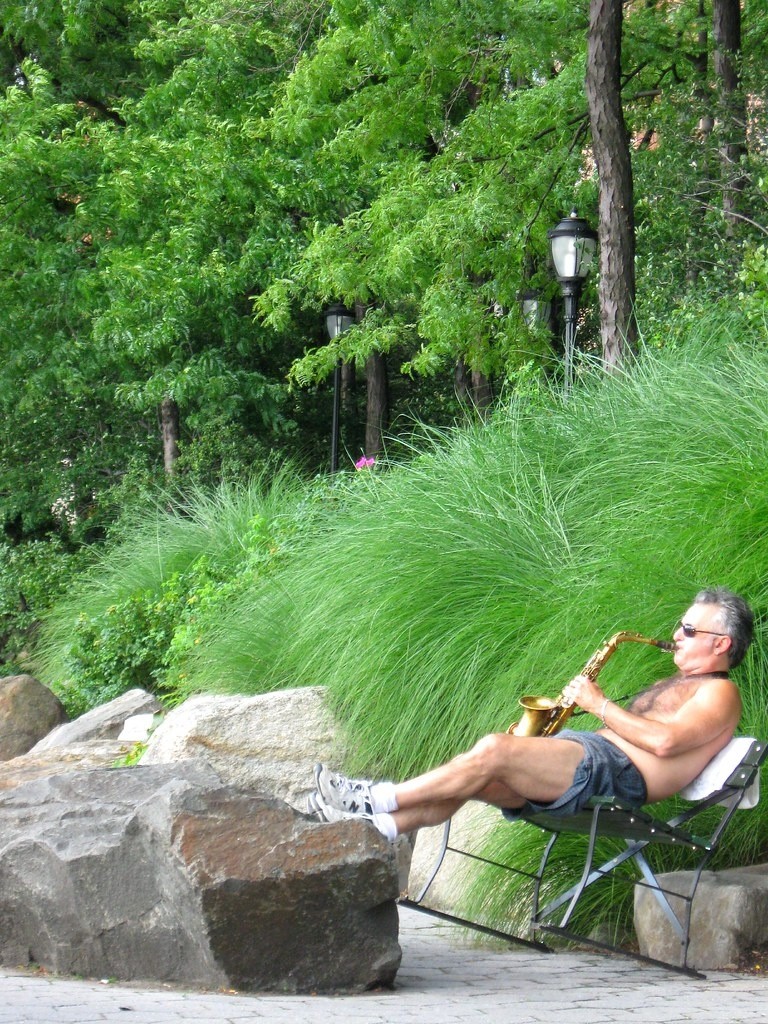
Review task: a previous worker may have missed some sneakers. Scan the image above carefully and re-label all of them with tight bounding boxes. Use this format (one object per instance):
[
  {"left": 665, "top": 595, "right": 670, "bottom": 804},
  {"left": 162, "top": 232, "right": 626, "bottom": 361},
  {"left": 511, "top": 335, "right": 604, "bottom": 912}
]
[
  {"left": 307, "top": 791, "right": 394, "bottom": 845},
  {"left": 313, "top": 763, "right": 373, "bottom": 815}
]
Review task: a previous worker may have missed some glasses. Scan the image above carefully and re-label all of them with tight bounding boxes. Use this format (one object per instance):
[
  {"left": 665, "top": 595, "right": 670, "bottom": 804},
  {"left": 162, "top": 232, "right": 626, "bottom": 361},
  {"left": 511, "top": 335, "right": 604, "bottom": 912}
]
[{"left": 678, "top": 621, "right": 723, "bottom": 638}]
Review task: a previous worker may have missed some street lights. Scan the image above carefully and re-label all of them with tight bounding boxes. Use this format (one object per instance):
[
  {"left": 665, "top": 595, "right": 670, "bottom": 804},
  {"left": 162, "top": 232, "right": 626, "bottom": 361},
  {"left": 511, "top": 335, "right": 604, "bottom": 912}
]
[
  {"left": 550, "top": 209, "right": 601, "bottom": 408},
  {"left": 324, "top": 298, "right": 359, "bottom": 474}
]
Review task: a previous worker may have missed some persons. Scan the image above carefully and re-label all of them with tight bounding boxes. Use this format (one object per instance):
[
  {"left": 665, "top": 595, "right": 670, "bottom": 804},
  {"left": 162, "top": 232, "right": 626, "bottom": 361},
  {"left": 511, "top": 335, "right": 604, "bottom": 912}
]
[{"left": 308, "top": 591, "right": 756, "bottom": 845}]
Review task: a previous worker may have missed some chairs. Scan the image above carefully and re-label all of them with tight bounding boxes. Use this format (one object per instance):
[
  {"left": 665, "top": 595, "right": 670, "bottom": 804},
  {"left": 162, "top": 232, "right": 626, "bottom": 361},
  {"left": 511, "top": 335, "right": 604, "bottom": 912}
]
[{"left": 395, "top": 737, "right": 767, "bottom": 982}]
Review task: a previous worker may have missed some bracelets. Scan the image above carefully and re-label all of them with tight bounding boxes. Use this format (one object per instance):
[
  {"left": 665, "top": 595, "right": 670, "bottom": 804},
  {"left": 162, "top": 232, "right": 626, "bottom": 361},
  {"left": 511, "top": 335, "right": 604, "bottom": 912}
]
[{"left": 601, "top": 699, "right": 610, "bottom": 729}]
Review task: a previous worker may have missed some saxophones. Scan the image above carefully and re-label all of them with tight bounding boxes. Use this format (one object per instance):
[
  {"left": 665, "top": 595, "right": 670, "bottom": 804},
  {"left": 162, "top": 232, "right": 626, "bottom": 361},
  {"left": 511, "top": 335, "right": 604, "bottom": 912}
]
[{"left": 504, "top": 630, "right": 679, "bottom": 739}]
